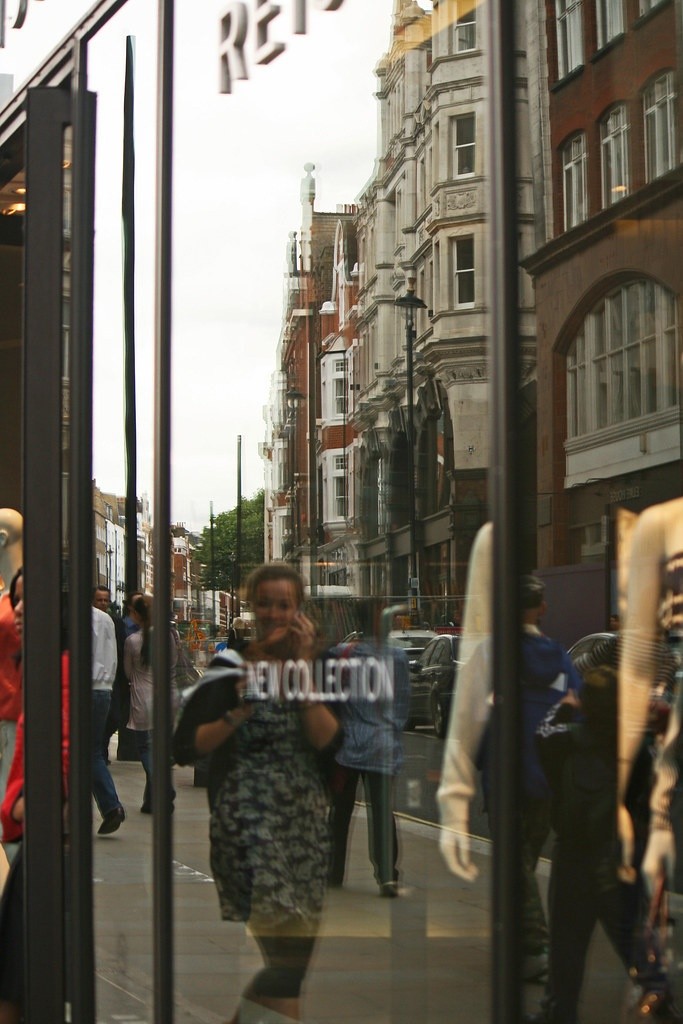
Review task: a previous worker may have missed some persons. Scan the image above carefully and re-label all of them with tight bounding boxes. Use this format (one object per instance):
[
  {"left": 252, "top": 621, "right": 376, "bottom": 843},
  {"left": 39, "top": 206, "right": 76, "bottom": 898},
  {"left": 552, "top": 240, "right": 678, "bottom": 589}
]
[
  {"left": 0, "top": 568, "right": 181, "bottom": 1024},
  {"left": 173, "top": 565, "right": 661, "bottom": 1023},
  {"left": 615, "top": 498, "right": 683, "bottom": 890},
  {"left": 0, "top": 507, "right": 25, "bottom": 859},
  {"left": 434, "top": 523, "right": 495, "bottom": 882}
]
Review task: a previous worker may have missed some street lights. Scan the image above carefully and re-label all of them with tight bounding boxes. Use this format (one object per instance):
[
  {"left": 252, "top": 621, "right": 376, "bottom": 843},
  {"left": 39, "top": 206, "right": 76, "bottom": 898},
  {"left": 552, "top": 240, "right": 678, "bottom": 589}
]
[{"left": 398, "top": 285, "right": 428, "bottom": 624}]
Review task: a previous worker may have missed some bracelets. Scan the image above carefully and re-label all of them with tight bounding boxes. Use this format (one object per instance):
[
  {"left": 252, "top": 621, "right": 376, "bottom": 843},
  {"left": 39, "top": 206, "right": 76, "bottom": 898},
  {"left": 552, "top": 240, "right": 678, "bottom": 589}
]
[{"left": 225, "top": 712, "right": 242, "bottom": 727}]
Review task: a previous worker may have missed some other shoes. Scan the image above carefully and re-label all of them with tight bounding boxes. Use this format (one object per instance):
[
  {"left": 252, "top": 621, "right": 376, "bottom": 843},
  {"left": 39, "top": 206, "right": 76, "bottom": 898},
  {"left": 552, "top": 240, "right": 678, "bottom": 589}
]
[
  {"left": 381, "top": 881, "right": 409, "bottom": 897},
  {"left": 97, "top": 806, "right": 125, "bottom": 834},
  {"left": 140, "top": 806, "right": 152, "bottom": 813}
]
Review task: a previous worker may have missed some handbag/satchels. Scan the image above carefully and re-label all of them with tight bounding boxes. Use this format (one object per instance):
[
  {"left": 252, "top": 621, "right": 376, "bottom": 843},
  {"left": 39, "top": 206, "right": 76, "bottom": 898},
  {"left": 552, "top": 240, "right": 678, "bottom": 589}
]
[
  {"left": 174, "top": 648, "right": 247, "bottom": 735},
  {"left": 171, "top": 628, "right": 205, "bottom": 691}
]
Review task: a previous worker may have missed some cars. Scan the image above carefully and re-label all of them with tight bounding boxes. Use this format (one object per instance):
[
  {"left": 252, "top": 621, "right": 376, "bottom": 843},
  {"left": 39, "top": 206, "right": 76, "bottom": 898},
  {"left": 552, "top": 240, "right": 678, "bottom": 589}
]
[
  {"left": 386, "top": 629, "right": 441, "bottom": 665},
  {"left": 403, "top": 634, "right": 462, "bottom": 738},
  {"left": 564, "top": 629, "right": 621, "bottom": 662}
]
